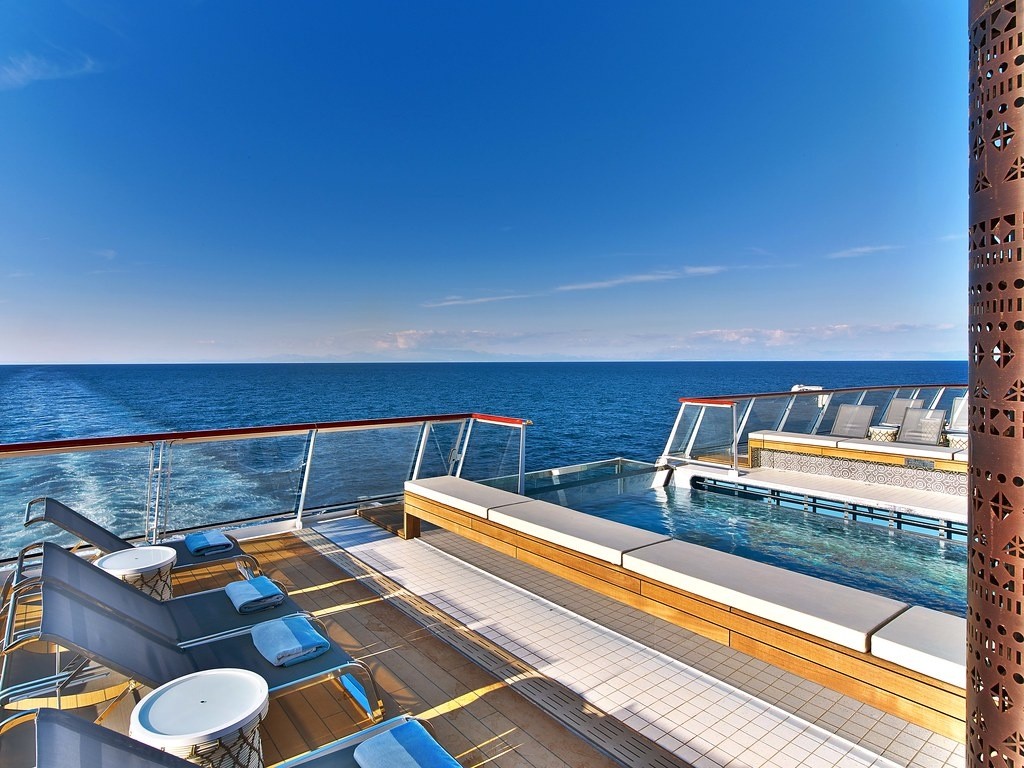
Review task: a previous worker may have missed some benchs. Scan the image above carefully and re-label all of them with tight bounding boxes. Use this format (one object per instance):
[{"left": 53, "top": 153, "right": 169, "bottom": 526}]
[
  {"left": 404, "top": 476, "right": 1011, "bottom": 758},
  {"left": 748, "top": 430, "right": 992, "bottom": 497}
]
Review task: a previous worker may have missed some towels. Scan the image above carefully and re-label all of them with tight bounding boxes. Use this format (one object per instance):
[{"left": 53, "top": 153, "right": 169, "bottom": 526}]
[
  {"left": 224, "top": 576, "right": 287, "bottom": 615},
  {"left": 250, "top": 616, "right": 331, "bottom": 668},
  {"left": 353, "top": 719, "right": 463, "bottom": 768},
  {"left": 183, "top": 530, "right": 234, "bottom": 557}
]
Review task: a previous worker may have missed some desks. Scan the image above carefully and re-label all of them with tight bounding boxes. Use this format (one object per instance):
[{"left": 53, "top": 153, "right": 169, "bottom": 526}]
[
  {"left": 129, "top": 669, "right": 269, "bottom": 768},
  {"left": 947, "top": 434, "right": 968, "bottom": 451},
  {"left": 93, "top": 546, "right": 177, "bottom": 602},
  {"left": 870, "top": 426, "right": 898, "bottom": 442}
]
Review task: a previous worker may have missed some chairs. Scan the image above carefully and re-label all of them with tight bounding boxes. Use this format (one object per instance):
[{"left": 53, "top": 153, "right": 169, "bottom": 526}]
[
  {"left": 892, "top": 407, "right": 949, "bottom": 447},
  {"left": 0, "top": 707, "right": 464, "bottom": 768},
  {"left": 24, "top": 496, "right": 263, "bottom": 580},
  {"left": 941, "top": 397, "right": 968, "bottom": 446},
  {"left": 823, "top": 403, "right": 878, "bottom": 439},
  {"left": 0, "top": 582, "right": 383, "bottom": 724},
  {"left": 0, "top": 541, "right": 318, "bottom": 693},
  {"left": 879, "top": 397, "right": 924, "bottom": 428}
]
